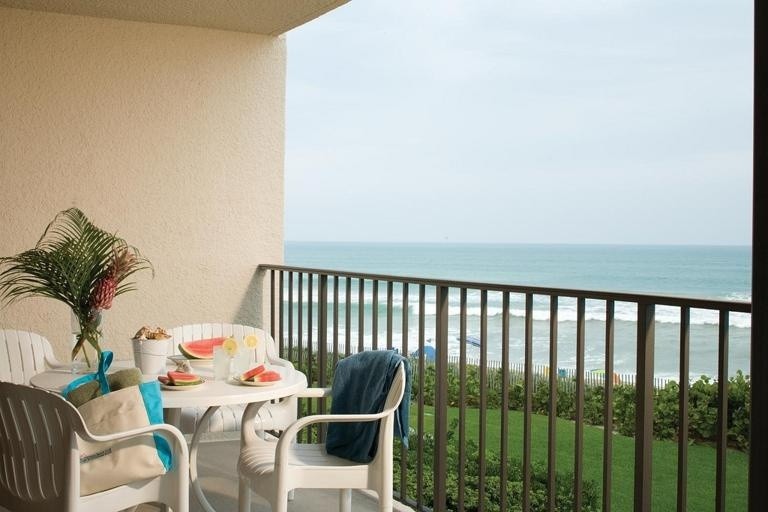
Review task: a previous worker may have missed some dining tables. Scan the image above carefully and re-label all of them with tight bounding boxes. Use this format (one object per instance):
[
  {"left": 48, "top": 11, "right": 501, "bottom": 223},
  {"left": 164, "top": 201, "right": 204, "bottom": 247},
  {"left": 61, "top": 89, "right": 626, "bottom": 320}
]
[{"left": 4, "top": 219, "right": 153, "bottom": 382}]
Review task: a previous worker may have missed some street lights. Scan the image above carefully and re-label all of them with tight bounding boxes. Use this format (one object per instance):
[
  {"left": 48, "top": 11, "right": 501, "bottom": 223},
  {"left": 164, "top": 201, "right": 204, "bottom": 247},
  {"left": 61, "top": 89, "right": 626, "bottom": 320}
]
[{"left": 61, "top": 351, "right": 174, "bottom": 498}]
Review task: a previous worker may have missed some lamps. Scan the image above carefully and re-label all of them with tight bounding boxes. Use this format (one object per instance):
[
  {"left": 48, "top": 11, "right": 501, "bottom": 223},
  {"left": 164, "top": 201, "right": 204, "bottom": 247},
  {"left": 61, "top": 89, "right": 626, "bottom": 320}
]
[
  {"left": 236, "top": 360, "right": 406, "bottom": 512},
  {"left": 1, "top": 330, "right": 90, "bottom": 383},
  {"left": 165, "top": 322, "right": 296, "bottom": 501},
  {"left": 0, "top": 382, "right": 192, "bottom": 511}
]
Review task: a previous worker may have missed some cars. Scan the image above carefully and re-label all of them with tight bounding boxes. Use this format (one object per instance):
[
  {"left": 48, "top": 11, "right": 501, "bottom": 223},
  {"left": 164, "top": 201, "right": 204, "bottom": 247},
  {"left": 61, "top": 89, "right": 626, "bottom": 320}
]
[
  {"left": 231, "top": 347, "right": 249, "bottom": 375},
  {"left": 213, "top": 345, "right": 230, "bottom": 380}
]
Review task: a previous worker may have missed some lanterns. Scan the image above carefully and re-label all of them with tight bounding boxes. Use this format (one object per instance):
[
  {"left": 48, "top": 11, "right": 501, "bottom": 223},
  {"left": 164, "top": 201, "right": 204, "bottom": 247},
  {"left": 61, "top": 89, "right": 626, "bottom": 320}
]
[
  {"left": 244, "top": 334, "right": 259, "bottom": 349},
  {"left": 222, "top": 338, "right": 239, "bottom": 353}
]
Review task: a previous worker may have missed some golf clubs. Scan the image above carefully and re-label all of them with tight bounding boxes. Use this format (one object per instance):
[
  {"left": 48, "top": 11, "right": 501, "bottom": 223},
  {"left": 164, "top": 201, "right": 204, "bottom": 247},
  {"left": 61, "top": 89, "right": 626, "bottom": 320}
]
[
  {"left": 167, "top": 371, "right": 201, "bottom": 385},
  {"left": 253, "top": 370, "right": 281, "bottom": 382},
  {"left": 239, "top": 365, "right": 266, "bottom": 381},
  {"left": 158, "top": 375, "right": 174, "bottom": 386},
  {"left": 178, "top": 337, "right": 231, "bottom": 359}
]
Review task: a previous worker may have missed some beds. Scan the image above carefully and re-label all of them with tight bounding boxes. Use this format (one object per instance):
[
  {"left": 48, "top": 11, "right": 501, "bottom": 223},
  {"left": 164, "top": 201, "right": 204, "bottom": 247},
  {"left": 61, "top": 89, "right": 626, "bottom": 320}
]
[{"left": 456, "top": 335, "right": 480, "bottom": 357}]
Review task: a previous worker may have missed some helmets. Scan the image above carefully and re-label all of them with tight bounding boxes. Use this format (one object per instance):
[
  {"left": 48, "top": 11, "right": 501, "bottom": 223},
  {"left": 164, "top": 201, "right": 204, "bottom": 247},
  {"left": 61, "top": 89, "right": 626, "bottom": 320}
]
[
  {"left": 159, "top": 377, "right": 205, "bottom": 390},
  {"left": 232, "top": 371, "right": 280, "bottom": 386},
  {"left": 169, "top": 355, "right": 221, "bottom": 368}
]
